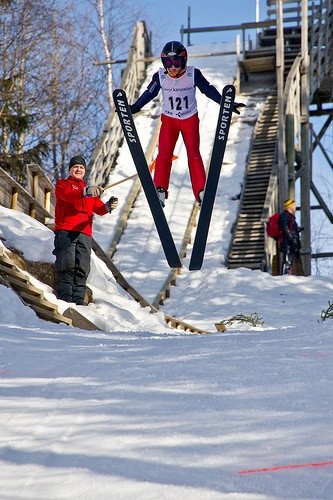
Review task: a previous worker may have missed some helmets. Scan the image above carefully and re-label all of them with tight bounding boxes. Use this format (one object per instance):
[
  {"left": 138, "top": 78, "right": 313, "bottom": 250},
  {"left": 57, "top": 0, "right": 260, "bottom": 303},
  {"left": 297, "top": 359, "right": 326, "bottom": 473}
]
[{"left": 160, "top": 41, "right": 188, "bottom": 76}]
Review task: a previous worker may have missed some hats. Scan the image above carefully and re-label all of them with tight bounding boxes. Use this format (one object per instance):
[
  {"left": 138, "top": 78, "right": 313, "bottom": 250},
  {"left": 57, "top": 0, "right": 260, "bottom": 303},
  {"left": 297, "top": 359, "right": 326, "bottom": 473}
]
[
  {"left": 69, "top": 156, "right": 86, "bottom": 171},
  {"left": 283, "top": 198, "right": 295, "bottom": 208}
]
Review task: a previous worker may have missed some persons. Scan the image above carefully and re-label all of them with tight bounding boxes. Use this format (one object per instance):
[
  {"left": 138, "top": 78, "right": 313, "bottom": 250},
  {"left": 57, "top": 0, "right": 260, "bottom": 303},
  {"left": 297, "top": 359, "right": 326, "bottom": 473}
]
[
  {"left": 52, "top": 156, "right": 118, "bottom": 306},
  {"left": 278, "top": 199, "right": 304, "bottom": 275},
  {"left": 115, "top": 41, "right": 247, "bottom": 207}
]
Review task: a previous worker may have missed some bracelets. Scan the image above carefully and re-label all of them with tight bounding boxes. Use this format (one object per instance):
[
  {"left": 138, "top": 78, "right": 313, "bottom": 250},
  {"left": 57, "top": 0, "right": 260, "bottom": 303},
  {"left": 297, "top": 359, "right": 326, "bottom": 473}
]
[{"left": 83, "top": 185, "right": 91, "bottom": 196}]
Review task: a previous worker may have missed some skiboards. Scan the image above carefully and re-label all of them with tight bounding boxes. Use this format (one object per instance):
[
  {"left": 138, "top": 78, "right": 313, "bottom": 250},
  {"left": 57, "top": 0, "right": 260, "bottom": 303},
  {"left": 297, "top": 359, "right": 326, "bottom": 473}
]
[{"left": 112, "top": 83, "right": 237, "bottom": 271}]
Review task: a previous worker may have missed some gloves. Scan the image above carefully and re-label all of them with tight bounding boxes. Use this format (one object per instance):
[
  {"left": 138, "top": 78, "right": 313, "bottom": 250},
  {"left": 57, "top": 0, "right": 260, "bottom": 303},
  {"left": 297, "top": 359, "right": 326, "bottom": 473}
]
[
  {"left": 84, "top": 185, "right": 105, "bottom": 197},
  {"left": 288, "top": 234, "right": 293, "bottom": 240},
  {"left": 105, "top": 196, "right": 118, "bottom": 214},
  {"left": 300, "top": 227, "right": 304, "bottom": 231},
  {"left": 128, "top": 105, "right": 141, "bottom": 114},
  {"left": 232, "top": 102, "right": 246, "bottom": 115}
]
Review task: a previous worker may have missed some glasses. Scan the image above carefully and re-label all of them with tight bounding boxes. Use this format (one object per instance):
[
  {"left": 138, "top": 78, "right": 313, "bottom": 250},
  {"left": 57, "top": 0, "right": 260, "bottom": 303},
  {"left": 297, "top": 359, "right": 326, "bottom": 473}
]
[{"left": 164, "top": 57, "right": 182, "bottom": 68}]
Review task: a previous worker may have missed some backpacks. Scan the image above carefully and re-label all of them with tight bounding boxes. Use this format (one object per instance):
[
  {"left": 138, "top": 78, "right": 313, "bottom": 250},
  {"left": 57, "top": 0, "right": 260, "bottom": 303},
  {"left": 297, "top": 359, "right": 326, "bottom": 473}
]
[{"left": 265, "top": 212, "right": 292, "bottom": 243}]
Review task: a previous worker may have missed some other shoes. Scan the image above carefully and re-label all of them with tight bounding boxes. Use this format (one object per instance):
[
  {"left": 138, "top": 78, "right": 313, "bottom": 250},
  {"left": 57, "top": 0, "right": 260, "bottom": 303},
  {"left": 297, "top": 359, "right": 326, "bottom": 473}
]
[
  {"left": 157, "top": 188, "right": 165, "bottom": 207},
  {"left": 199, "top": 190, "right": 205, "bottom": 206}
]
